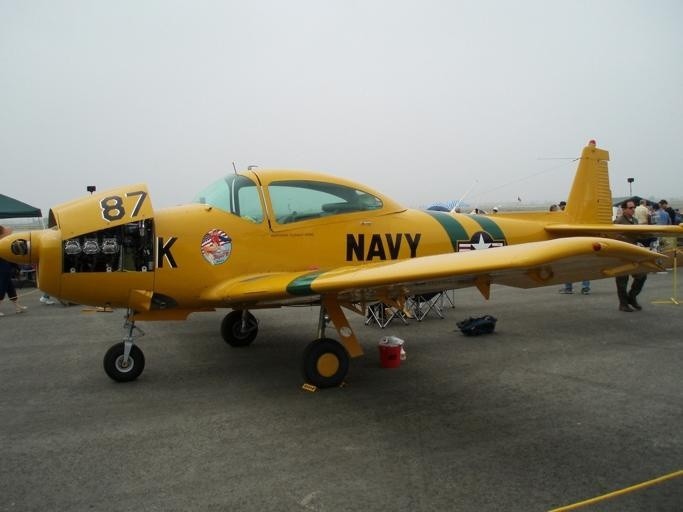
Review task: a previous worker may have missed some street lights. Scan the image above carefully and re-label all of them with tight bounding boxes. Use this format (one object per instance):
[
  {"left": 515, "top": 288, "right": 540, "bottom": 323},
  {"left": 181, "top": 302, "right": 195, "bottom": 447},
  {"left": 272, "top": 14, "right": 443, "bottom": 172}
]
[{"left": 628, "top": 178, "right": 634, "bottom": 197}]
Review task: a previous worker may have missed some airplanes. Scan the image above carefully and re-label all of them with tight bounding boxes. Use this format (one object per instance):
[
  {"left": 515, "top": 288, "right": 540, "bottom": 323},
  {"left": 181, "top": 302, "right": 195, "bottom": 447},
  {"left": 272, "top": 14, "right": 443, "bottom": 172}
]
[{"left": 0, "top": 139, "right": 683, "bottom": 389}]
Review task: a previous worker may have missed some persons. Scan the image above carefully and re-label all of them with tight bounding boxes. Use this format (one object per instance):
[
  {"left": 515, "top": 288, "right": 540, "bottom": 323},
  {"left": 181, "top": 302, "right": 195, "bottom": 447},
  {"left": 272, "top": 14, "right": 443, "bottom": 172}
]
[
  {"left": 612, "top": 199, "right": 683, "bottom": 312},
  {"left": 0, "top": 225, "right": 57, "bottom": 317},
  {"left": 455, "top": 206, "right": 499, "bottom": 214},
  {"left": 368, "top": 291, "right": 443, "bottom": 323},
  {"left": 559, "top": 279, "right": 594, "bottom": 295},
  {"left": 549, "top": 200, "right": 566, "bottom": 211}
]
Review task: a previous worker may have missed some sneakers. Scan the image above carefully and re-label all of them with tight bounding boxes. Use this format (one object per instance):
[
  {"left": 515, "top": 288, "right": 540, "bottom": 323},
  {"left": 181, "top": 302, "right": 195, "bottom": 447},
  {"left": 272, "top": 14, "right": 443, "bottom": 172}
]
[{"left": 560, "top": 288, "right": 642, "bottom": 312}]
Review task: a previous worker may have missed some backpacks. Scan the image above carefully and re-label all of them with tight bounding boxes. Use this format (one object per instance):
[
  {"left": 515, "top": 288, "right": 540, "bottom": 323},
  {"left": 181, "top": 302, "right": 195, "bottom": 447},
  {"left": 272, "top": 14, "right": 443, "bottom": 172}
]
[{"left": 455, "top": 314, "right": 497, "bottom": 336}]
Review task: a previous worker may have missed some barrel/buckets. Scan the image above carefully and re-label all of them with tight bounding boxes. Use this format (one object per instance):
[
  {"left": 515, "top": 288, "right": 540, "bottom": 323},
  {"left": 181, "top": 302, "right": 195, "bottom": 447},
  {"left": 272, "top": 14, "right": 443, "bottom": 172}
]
[{"left": 377, "top": 343, "right": 401, "bottom": 366}]
